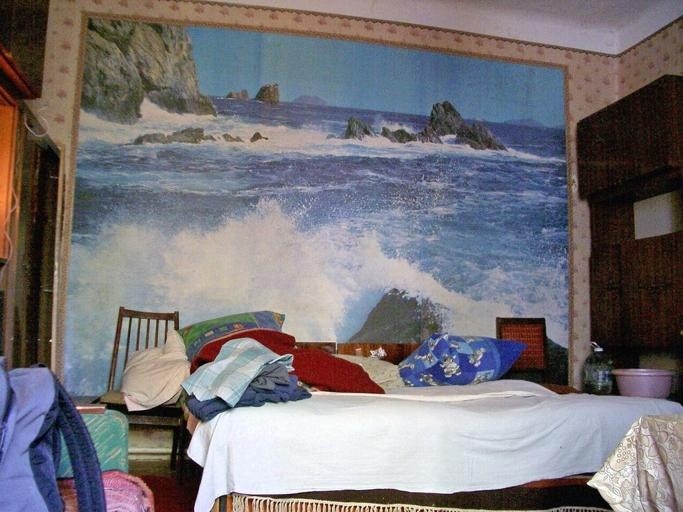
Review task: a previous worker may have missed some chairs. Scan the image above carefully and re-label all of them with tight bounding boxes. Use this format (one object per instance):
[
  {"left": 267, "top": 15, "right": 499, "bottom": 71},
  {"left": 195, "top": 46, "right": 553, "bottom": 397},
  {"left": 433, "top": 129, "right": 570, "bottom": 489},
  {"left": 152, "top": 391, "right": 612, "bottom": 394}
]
[
  {"left": 495, "top": 316, "right": 578, "bottom": 396},
  {"left": 99, "top": 305, "right": 186, "bottom": 478},
  {"left": 39, "top": 408, "right": 156, "bottom": 512}
]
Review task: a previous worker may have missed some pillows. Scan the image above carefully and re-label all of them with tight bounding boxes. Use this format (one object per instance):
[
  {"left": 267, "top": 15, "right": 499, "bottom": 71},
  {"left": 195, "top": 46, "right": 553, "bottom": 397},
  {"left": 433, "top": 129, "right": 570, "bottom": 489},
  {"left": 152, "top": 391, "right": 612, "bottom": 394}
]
[
  {"left": 400, "top": 332, "right": 526, "bottom": 385},
  {"left": 330, "top": 354, "right": 401, "bottom": 386},
  {"left": 176, "top": 309, "right": 296, "bottom": 359},
  {"left": 273, "top": 343, "right": 385, "bottom": 394}
]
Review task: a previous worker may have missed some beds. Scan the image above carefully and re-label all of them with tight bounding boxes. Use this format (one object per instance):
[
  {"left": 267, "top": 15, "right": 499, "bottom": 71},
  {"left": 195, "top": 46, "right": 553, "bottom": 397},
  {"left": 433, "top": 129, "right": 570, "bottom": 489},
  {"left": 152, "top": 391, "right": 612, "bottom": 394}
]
[{"left": 191, "top": 342, "right": 683, "bottom": 512}]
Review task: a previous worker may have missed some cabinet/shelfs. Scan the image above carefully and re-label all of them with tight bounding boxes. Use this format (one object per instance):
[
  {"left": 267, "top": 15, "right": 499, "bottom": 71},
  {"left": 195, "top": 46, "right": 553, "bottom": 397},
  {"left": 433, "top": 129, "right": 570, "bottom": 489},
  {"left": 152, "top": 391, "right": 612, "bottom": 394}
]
[
  {"left": 576, "top": 73, "right": 682, "bottom": 404},
  {"left": 0, "top": 0, "right": 60, "bottom": 374}
]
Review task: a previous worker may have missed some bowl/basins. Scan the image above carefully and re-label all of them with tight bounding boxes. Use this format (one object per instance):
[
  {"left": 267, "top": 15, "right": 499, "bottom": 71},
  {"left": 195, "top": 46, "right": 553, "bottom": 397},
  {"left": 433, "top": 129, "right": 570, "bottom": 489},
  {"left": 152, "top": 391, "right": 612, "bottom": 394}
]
[{"left": 611, "top": 369, "right": 676, "bottom": 399}]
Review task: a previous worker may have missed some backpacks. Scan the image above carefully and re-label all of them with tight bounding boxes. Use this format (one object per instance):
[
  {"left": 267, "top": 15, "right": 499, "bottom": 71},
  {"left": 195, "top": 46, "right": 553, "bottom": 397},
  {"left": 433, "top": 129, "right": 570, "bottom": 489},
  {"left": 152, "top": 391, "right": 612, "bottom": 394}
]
[{"left": 1, "top": 355, "right": 106, "bottom": 512}]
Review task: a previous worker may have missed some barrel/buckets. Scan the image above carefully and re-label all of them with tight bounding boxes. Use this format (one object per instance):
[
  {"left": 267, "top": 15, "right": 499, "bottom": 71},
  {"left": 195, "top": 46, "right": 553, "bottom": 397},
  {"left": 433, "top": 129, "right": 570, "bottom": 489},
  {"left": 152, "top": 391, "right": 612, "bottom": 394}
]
[{"left": 582, "top": 342, "right": 613, "bottom": 395}]
